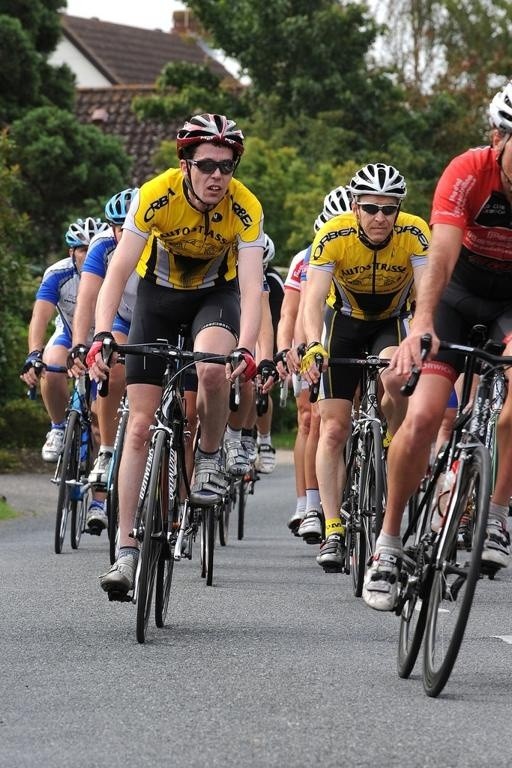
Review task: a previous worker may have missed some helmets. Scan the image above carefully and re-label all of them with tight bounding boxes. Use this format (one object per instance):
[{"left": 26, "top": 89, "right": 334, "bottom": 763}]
[
  {"left": 64, "top": 216, "right": 112, "bottom": 248},
  {"left": 313, "top": 212, "right": 327, "bottom": 237},
  {"left": 103, "top": 187, "right": 139, "bottom": 227},
  {"left": 263, "top": 232, "right": 276, "bottom": 265},
  {"left": 349, "top": 161, "right": 408, "bottom": 200},
  {"left": 175, "top": 113, "right": 244, "bottom": 158},
  {"left": 321, "top": 185, "right": 352, "bottom": 222},
  {"left": 488, "top": 80, "right": 512, "bottom": 134}
]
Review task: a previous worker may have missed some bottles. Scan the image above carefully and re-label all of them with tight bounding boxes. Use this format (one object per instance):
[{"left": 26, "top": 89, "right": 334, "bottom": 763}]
[{"left": 431, "top": 460, "right": 459, "bottom": 535}]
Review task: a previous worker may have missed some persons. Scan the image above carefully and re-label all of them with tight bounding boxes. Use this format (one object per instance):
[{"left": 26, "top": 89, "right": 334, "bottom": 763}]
[{"left": 20, "top": 80, "right": 512, "bottom": 611}]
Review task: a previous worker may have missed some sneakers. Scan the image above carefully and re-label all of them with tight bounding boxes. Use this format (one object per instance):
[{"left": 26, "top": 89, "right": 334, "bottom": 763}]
[
  {"left": 472, "top": 512, "right": 511, "bottom": 569},
  {"left": 361, "top": 545, "right": 405, "bottom": 611},
  {"left": 87, "top": 450, "right": 113, "bottom": 483},
  {"left": 85, "top": 506, "right": 108, "bottom": 533},
  {"left": 41, "top": 427, "right": 75, "bottom": 462},
  {"left": 98, "top": 553, "right": 138, "bottom": 592},
  {"left": 240, "top": 439, "right": 257, "bottom": 463},
  {"left": 256, "top": 442, "right": 277, "bottom": 474},
  {"left": 316, "top": 531, "right": 347, "bottom": 569},
  {"left": 223, "top": 430, "right": 250, "bottom": 475},
  {"left": 287, "top": 508, "right": 305, "bottom": 530},
  {"left": 189, "top": 452, "right": 230, "bottom": 506},
  {"left": 298, "top": 509, "right": 323, "bottom": 543}
]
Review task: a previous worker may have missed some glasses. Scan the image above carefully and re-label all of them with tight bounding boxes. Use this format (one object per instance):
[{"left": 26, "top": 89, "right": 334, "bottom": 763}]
[
  {"left": 184, "top": 156, "right": 235, "bottom": 175},
  {"left": 356, "top": 200, "right": 399, "bottom": 216}
]
